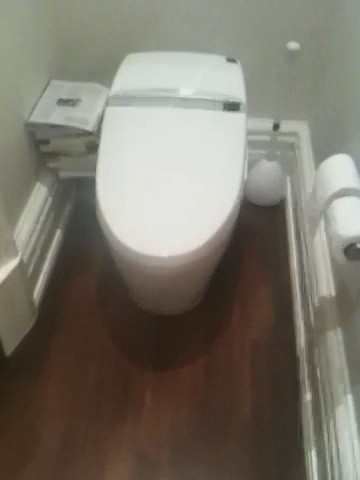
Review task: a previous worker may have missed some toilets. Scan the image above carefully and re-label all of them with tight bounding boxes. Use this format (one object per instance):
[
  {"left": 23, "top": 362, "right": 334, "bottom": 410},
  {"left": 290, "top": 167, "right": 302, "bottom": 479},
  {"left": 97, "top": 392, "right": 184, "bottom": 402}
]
[{"left": 94, "top": 51, "right": 252, "bottom": 316}]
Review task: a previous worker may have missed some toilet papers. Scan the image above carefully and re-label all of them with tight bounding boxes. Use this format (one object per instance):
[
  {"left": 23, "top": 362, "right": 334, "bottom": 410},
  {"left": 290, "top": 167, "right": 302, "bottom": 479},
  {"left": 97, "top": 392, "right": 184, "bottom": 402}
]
[{"left": 317, "top": 152, "right": 360, "bottom": 264}]
